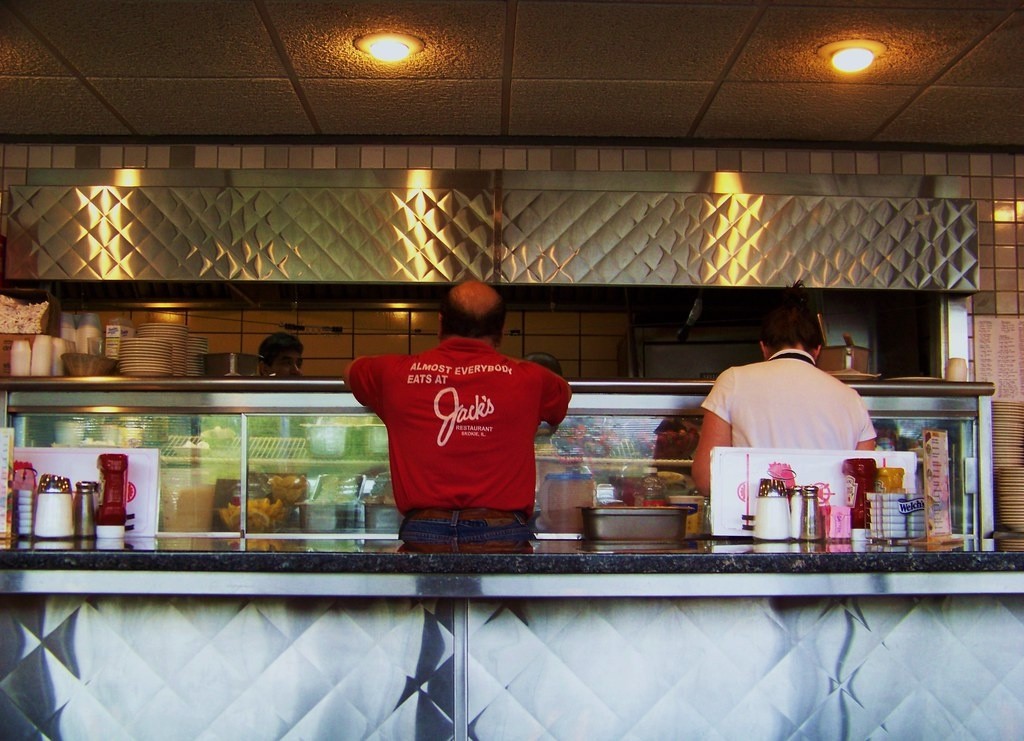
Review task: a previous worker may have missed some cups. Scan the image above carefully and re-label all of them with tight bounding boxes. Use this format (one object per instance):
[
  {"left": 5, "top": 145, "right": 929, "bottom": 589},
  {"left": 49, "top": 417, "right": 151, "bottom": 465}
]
[
  {"left": 61, "top": 313, "right": 102, "bottom": 353},
  {"left": 875, "top": 468, "right": 904, "bottom": 493}
]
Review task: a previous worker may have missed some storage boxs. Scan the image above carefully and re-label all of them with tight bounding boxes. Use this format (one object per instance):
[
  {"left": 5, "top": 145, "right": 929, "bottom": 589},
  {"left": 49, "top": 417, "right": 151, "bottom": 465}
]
[
  {"left": 105, "top": 325, "right": 136, "bottom": 360},
  {"left": 0, "top": 332, "right": 43, "bottom": 377}
]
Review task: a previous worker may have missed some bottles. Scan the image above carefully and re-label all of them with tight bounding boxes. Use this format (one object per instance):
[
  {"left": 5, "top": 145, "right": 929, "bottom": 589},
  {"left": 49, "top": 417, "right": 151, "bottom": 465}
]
[
  {"left": 800, "top": 485, "right": 823, "bottom": 540},
  {"left": 789, "top": 486, "right": 803, "bottom": 540},
  {"left": 842, "top": 459, "right": 877, "bottom": 540},
  {"left": 74, "top": 481, "right": 99, "bottom": 538},
  {"left": 751, "top": 478, "right": 790, "bottom": 540},
  {"left": 634, "top": 467, "right": 667, "bottom": 507},
  {"left": 9, "top": 335, "right": 77, "bottom": 376},
  {"left": 96, "top": 454, "right": 130, "bottom": 538}
]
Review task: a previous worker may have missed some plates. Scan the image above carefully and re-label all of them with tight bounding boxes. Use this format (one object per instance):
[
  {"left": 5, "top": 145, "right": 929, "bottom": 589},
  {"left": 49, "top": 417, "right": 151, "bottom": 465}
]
[
  {"left": 991, "top": 401, "right": 1023, "bottom": 525},
  {"left": 995, "top": 540, "right": 1024, "bottom": 553},
  {"left": 119, "top": 323, "right": 208, "bottom": 375}
]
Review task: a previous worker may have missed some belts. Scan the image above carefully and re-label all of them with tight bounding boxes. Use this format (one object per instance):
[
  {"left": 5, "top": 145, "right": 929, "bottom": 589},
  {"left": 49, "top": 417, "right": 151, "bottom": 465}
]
[{"left": 408, "top": 508, "right": 518, "bottom": 521}]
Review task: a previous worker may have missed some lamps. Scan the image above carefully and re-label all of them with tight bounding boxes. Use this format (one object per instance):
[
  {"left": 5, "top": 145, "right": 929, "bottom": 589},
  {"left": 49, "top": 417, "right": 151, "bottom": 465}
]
[
  {"left": 817, "top": 39, "right": 887, "bottom": 72},
  {"left": 354, "top": 32, "right": 427, "bottom": 63}
]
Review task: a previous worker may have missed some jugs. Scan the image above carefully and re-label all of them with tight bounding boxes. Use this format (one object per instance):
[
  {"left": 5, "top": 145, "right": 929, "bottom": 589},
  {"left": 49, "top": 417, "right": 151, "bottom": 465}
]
[
  {"left": 34, "top": 473, "right": 73, "bottom": 538},
  {"left": 538, "top": 474, "right": 594, "bottom": 533}
]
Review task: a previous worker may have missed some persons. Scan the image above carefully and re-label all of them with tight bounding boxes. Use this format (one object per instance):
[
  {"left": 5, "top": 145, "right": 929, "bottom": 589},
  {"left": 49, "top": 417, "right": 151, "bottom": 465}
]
[
  {"left": 691, "top": 279, "right": 877, "bottom": 497},
  {"left": 523, "top": 351, "right": 563, "bottom": 376},
  {"left": 258, "top": 331, "right": 304, "bottom": 376},
  {"left": 343, "top": 278, "right": 572, "bottom": 557}
]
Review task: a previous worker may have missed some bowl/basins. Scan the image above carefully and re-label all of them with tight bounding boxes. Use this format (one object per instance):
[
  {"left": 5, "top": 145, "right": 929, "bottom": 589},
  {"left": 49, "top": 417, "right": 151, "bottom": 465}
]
[
  {"left": 204, "top": 352, "right": 264, "bottom": 378},
  {"left": 60, "top": 353, "right": 119, "bottom": 377}
]
